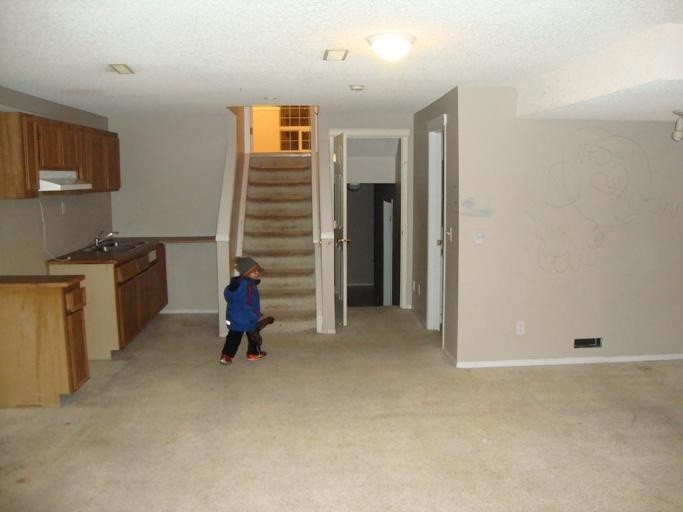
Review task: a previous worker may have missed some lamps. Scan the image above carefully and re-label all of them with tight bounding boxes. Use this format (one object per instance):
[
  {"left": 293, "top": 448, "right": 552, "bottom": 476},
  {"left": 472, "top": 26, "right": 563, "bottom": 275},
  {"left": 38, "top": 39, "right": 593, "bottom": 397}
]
[
  {"left": 672, "top": 111, "right": 683, "bottom": 142},
  {"left": 348, "top": 183, "right": 360, "bottom": 191}
]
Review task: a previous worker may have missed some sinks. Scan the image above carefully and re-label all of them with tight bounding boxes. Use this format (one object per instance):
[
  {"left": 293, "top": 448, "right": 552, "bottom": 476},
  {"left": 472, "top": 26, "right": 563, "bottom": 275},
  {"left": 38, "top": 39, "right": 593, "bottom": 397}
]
[
  {"left": 108, "top": 239, "right": 144, "bottom": 245},
  {"left": 96, "top": 245, "right": 135, "bottom": 252}
]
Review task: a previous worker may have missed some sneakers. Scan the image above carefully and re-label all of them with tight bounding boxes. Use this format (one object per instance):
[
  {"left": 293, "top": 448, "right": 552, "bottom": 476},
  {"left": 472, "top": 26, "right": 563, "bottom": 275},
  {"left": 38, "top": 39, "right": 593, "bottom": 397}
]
[
  {"left": 220, "top": 355, "right": 232, "bottom": 363},
  {"left": 247, "top": 352, "right": 266, "bottom": 360}
]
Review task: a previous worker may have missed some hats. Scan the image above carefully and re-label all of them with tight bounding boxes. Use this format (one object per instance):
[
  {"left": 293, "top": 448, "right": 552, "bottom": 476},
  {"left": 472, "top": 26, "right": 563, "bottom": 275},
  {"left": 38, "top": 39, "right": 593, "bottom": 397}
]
[{"left": 235, "top": 257, "right": 257, "bottom": 275}]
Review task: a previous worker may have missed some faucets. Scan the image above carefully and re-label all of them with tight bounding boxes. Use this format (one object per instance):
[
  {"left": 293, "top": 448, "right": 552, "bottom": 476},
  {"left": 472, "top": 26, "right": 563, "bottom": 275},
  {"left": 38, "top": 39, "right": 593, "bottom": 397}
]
[{"left": 95, "top": 229, "right": 119, "bottom": 247}]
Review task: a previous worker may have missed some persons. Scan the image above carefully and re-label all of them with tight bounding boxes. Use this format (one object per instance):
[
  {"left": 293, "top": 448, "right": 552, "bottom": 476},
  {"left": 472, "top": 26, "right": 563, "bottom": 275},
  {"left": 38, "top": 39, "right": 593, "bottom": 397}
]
[{"left": 220, "top": 256, "right": 266, "bottom": 364}]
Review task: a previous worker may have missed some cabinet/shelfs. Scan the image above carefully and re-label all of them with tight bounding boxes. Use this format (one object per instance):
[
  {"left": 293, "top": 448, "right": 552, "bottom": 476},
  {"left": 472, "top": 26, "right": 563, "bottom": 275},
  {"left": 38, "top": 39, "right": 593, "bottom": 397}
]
[
  {"left": 0, "top": 112, "right": 120, "bottom": 199},
  {"left": 0, "top": 245, "right": 168, "bottom": 408}
]
